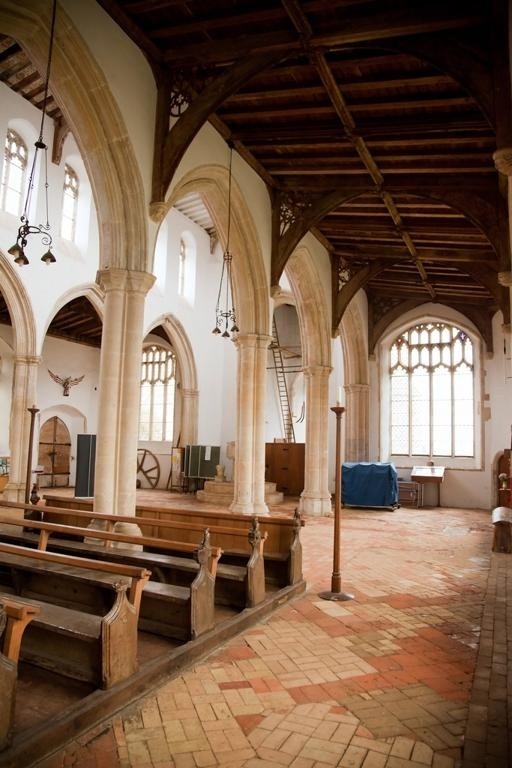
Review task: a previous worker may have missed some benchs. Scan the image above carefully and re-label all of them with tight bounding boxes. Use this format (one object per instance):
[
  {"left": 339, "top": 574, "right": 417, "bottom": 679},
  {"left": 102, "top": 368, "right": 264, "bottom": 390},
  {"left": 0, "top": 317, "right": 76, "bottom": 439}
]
[{"left": 2, "top": 495, "right": 302, "bottom": 728}]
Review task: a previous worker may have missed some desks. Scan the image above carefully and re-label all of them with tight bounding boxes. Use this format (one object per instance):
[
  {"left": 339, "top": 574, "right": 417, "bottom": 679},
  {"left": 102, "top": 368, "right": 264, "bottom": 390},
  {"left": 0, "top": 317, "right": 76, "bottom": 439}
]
[{"left": 410, "top": 465, "right": 444, "bottom": 505}]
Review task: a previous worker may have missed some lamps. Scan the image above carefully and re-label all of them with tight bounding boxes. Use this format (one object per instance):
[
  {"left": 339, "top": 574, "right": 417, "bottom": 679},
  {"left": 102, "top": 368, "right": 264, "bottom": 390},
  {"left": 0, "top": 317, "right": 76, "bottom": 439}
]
[
  {"left": 212, "top": 140, "right": 240, "bottom": 339},
  {"left": 2, "top": 1, "right": 60, "bottom": 266}
]
